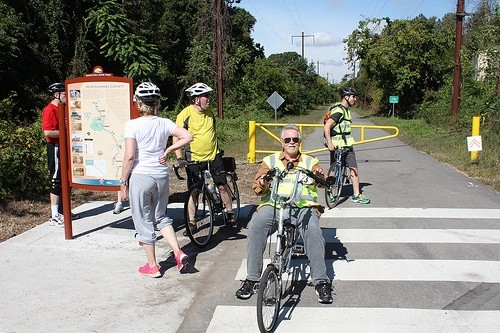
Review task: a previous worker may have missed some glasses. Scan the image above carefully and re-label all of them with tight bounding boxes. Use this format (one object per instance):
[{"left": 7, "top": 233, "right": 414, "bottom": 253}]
[
  {"left": 284, "top": 137, "right": 299, "bottom": 143},
  {"left": 350, "top": 95, "right": 356, "bottom": 98}
]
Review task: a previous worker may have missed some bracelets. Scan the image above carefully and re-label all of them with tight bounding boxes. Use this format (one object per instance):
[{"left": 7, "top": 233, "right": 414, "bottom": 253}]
[{"left": 119, "top": 178, "right": 126, "bottom": 185}]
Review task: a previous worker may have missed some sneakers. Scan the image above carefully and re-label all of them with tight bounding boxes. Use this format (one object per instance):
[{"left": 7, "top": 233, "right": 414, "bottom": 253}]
[
  {"left": 235, "top": 278, "right": 260, "bottom": 300},
  {"left": 327, "top": 191, "right": 335, "bottom": 203},
  {"left": 315, "top": 282, "right": 333, "bottom": 304},
  {"left": 351, "top": 193, "right": 370, "bottom": 204},
  {"left": 174, "top": 249, "right": 190, "bottom": 274},
  {"left": 49, "top": 213, "right": 64, "bottom": 227},
  {"left": 183, "top": 221, "right": 197, "bottom": 236},
  {"left": 112, "top": 202, "right": 124, "bottom": 214},
  {"left": 71, "top": 212, "right": 80, "bottom": 220},
  {"left": 225, "top": 212, "right": 237, "bottom": 226},
  {"left": 138, "top": 263, "right": 162, "bottom": 278}
]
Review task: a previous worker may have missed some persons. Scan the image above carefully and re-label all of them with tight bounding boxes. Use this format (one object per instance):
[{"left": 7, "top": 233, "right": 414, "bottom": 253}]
[
  {"left": 121, "top": 81, "right": 188, "bottom": 278},
  {"left": 113, "top": 95, "right": 145, "bottom": 214},
  {"left": 235, "top": 126, "right": 333, "bottom": 303},
  {"left": 172, "top": 83, "right": 235, "bottom": 236},
  {"left": 41, "top": 83, "right": 80, "bottom": 227},
  {"left": 324, "top": 87, "right": 370, "bottom": 204}
]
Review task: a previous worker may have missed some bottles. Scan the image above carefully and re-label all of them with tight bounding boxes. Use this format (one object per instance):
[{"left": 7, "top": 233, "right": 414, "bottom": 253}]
[{"left": 206, "top": 183, "right": 217, "bottom": 201}]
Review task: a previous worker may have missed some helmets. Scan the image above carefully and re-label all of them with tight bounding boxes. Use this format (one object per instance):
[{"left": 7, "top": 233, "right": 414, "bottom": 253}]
[
  {"left": 185, "top": 82, "right": 214, "bottom": 98},
  {"left": 134, "top": 81, "right": 162, "bottom": 103},
  {"left": 45, "top": 82, "right": 65, "bottom": 96},
  {"left": 339, "top": 87, "right": 359, "bottom": 97}
]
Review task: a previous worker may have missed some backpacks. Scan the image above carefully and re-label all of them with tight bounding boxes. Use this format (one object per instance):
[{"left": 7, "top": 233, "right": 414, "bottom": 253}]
[{"left": 323, "top": 104, "right": 352, "bottom": 138}]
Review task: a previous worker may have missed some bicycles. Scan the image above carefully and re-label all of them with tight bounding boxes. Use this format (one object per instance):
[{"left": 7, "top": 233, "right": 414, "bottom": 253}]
[
  {"left": 320, "top": 141, "right": 352, "bottom": 208},
  {"left": 255, "top": 162, "right": 336, "bottom": 333},
  {"left": 173, "top": 147, "right": 241, "bottom": 246}
]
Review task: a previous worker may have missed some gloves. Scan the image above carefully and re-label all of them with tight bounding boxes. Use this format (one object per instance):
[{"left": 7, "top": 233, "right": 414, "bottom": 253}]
[{"left": 177, "top": 156, "right": 188, "bottom": 169}]
